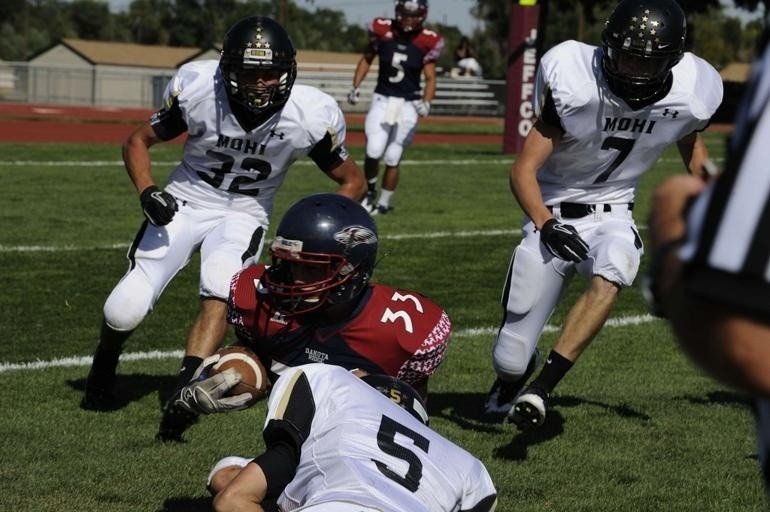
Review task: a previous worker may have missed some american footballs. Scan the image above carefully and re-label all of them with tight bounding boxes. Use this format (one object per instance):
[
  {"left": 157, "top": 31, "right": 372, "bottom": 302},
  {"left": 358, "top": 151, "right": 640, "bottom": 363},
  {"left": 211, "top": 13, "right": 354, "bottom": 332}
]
[{"left": 208, "top": 346, "right": 267, "bottom": 408}]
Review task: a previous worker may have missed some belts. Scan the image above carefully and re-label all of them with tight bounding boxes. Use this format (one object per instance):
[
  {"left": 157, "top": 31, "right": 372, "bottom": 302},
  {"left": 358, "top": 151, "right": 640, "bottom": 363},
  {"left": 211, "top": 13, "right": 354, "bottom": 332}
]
[{"left": 546, "top": 201, "right": 634, "bottom": 218}]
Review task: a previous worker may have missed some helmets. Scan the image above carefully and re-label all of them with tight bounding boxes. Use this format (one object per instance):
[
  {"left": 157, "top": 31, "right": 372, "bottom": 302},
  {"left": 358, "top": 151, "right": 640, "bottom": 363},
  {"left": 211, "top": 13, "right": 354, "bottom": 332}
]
[
  {"left": 255, "top": 193, "right": 378, "bottom": 326},
  {"left": 360, "top": 374, "right": 429, "bottom": 427},
  {"left": 221, "top": 16, "right": 297, "bottom": 115},
  {"left": 601, "top": 0, "right": 687, "bottom": 103},
  {"left": 395, "top": 0, "right": 428, "bottom": 33}
]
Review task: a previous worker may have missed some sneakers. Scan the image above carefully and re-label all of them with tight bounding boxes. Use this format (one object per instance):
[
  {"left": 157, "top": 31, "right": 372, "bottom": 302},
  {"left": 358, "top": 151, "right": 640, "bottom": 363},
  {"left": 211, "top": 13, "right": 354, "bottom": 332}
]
[
  {"left": 507, "top": 387, "right": 549, "bottom": 432},
  {"left": 369, "top": 203, "right": 389, "bottom": 216},
  {"left": 84, "top": 344, "right": 120, "bottom": 410},
  {"left": 360, "top": 189, "right": 377, "bottom": 213},
  {"left": 483, "top": 348, "right": 541, "bottom": 415}
]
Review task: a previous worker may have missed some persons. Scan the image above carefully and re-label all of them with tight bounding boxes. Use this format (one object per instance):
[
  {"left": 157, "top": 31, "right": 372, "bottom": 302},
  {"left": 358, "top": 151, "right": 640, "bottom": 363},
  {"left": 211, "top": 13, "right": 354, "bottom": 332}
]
[
  {"left": 347, "top": 0, "right": 446, "bottom": 217},
  {"left": 646, "top": 29, "right": 769, "bottom": 410},
  {"left": 451, "top": 37, "right": 486, "bottom": 79},
  {"left": 179, "top": 193, "right": 454, "bottom": 414},
  {"left": 198, "top": 361, "right": 500, "bottom": 512},
  {"left": 481, "top": 0, "right": 725, "bottom": 431},
  {"left": 82, "top": 15, "right": 369, "bottom": 420}
]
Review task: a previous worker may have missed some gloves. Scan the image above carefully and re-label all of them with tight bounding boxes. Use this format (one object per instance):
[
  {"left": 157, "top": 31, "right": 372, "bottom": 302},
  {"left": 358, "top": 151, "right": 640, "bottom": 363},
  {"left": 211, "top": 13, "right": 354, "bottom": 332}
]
[
  {"left": 139, "top": 184, "right": 179, "bottom": 227},
  {"left": 412, "top": 98, "right": 431, "bottom": 118},
  {"left": 540, "top": 219, "right": 590, "bottom": 264},
  {"left": 348, "top": 88, "right": 360, "bottom": 105},
  {"left": 174, "top": 354, "right": 253, "bottom": 417}
]
